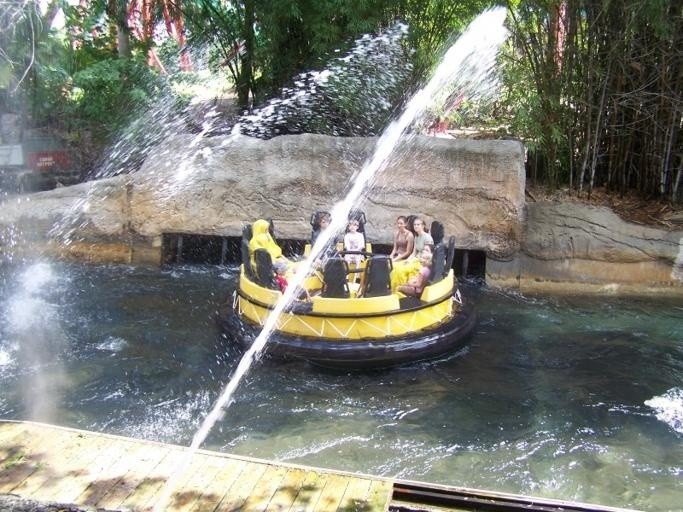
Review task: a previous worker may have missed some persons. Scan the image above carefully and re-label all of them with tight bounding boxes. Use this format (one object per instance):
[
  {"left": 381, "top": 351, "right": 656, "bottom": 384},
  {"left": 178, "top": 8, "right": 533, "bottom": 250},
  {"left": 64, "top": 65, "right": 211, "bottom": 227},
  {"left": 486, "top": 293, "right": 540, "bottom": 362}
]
[
  {"left": 273, "top": 261, "right": 289, "bottom": 291},
  {"left": 400, "top": 217, "right": 434, "bottom": 281},
  {"left": 343, "top": 219, "right": 365, "bottom": 285},
  {"left": 247, "top": 219, "right": 282, "bottom": 273},
  {"left": 388, "top": 215, "right": 415, "bottom": 262},
  {"left": 397, "top": 243, "right": 433, "bottom": 297},
  {"left": 310, "top": 211, "right": 337, "bottom": 259}
]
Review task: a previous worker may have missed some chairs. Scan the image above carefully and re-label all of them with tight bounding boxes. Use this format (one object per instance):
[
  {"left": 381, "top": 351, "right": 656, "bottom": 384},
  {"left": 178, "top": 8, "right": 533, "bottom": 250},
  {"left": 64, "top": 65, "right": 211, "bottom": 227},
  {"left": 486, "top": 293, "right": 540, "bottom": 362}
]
[{"left": 241, "top": 210, "right": 455, "bottom": 297}]
